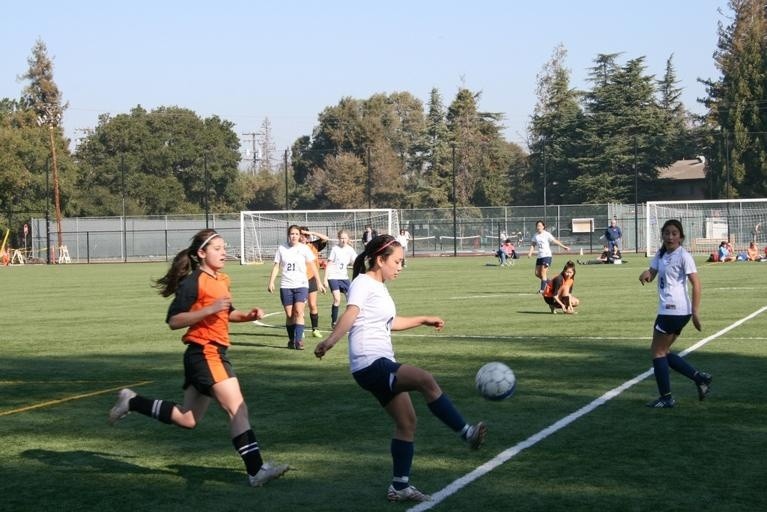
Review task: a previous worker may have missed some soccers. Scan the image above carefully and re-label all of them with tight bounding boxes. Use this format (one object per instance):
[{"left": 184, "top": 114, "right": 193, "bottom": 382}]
[{"left": 474, "top": 361, "right": 516, "bottom": 400}]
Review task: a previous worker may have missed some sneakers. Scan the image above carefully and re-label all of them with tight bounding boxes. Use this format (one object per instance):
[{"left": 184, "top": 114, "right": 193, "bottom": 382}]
[
  {"left": 109, "top": 387, "right": 137, "bottom": 423},
  {"left": 248, "top": 461, "right": 290, "bottom": 486},
  {"left": 288, "top": 321, "right": 337, "bottom": 350},
  {"left": 696, "top": 371, "right": 712, "bottom": 401},
  {"left": 388, "top": 485, "right": 433, "bottom": 503},
  {"left": 469, "top": 420, "right": 488, "bottom": 451},
  {"left": 646, "top": 397, "right": 674, "bottom": 409}
]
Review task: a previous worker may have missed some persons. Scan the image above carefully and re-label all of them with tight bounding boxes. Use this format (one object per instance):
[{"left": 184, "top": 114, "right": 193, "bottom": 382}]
[
  {"left": 295, "top": 226, "right": 329, "bottom": 339},
  {"left": 395, "top": 230, "right": 408, "bottom": 268},
  {"left": 497, "top": 239, "right": 516, "bottom": 267},
  {"left": 477, "top": 225, "right": 488, "bottom": 248},
  {"left": 430, "top": 227, "right": 443, "bottom": 251},
  {"left": 314, "top": 232, "right": 489, "bottom": 507},
  {"left": 542, "top": 259, "right": 580, "bottom": 314},
  {"left": 403, "top": 229, "right": 411, "bottom": 252},
  {"left": 499, "top": 231, "right": 508, "bottom": 246},
  {"left": 527, "top": 219, "right": 570, "bottom": 294},
  {"left": 723, "top": 242, "right": 734, "bottom": 256},
  {"left": 516, "top": 228, "right": 526, "bottom": 248},
  {"left": 577, "top": 244, "right": 610, "bottom": 265},
  {"left": 321, "top": 229, "right": 358, "bottom": 329},
  {"left": 637, "top": 218, "right": 712, "bottom": 408},
  {"left": 107, "top": 227, "right": 293, "bottom": 489},
  {"left": 745, "top": 241, "right": 762, "bottom": 262},
  {"left": 604, "top": 220, "right": 622, "bottom": 254},
  {"left": 718, "top": 240, "right": 737, "bottom": 263},
  {"left": 361, "top": 225, "right": 379, "bottom": 249},
  {"left": 608, "top": 244, "right": 623, "bottom": 265},
  {"left": 267, "top": 224, "right": 327, "bottom": 350}
]
